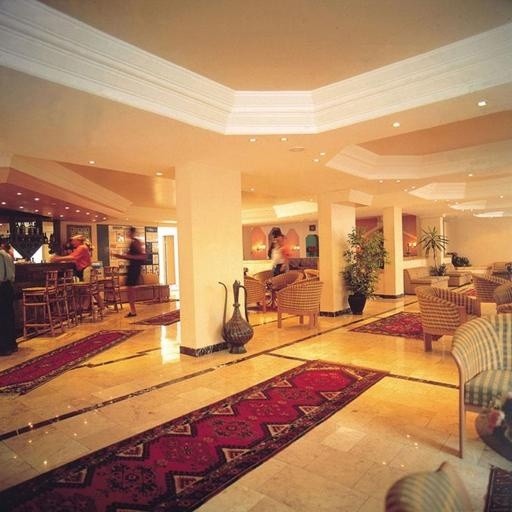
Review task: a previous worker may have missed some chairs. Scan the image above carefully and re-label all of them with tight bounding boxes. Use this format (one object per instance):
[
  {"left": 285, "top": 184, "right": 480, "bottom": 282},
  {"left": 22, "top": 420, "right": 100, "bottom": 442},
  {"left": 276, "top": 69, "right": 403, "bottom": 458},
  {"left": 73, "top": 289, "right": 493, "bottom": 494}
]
[
  {"left": 444, "top": 263, "right": 472, "bottom": 287},
  {"left": 386, "top": 462, "right": 474, "bottom": 512},
  {"left": 472, "top": 273, "right": 511, "bottom": 303},
  {"left": 244, "top": 270, "right": 325, "bottom": 330},
  {"left": 495, "top": 283, "right": 512, "bottom": 314},
  {"left": 490, "top": 262, "right": 507, "bottom": 275},
  {"left": 414, "top": 286, "right": 481, "bottom": 353},
  {"left": 403, "top": 267, "right": 449, "bottom": 295},
  {"left": 21, "top": 266, "right": 122, "bottom": 338},
  {"left": 449, "top": 312, "right": 512, "bottom": 459}
]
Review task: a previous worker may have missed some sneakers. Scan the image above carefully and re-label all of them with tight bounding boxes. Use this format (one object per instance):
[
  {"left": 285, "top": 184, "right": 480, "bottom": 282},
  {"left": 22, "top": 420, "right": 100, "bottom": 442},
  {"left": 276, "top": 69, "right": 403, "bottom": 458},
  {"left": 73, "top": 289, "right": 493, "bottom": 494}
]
[
  {"left": 125, "top": 313, "right": 138, "bottom": 318},
  {"left": 1, "top": 342, "right": 18, "bottom": 356}
]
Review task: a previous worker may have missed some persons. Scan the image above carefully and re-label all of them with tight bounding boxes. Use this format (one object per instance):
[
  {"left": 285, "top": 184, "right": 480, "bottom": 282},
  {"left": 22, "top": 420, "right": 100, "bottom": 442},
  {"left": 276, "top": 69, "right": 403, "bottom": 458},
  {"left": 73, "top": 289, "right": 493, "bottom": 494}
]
[
  {"left": 114, "top": 226, "right": 147, "bottom": 317},
  {"left": 52, "top": 234, "right": 106, "bottom": 312},
  {"left": 1, "top": 238, "right": 19, "bottom": 356},
  {"left": 271, "top": 237, "right": 290, "bottom": 279}
]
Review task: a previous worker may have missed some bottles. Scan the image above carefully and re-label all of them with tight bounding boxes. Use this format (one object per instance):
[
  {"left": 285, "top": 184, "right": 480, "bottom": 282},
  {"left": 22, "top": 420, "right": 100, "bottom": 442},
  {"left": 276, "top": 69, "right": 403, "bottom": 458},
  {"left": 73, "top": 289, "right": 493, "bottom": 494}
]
[
  {"left": 30, "top": 257, "right": 35, "bottom": 264},
  {"left": 13, "top": 220, "right": 55, "bottom": 243}
]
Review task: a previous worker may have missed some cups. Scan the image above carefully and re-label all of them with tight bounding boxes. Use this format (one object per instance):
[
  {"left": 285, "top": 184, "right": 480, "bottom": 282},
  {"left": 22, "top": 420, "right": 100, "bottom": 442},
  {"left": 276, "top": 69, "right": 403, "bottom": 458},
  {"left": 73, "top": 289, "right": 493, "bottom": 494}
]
[{"left": 41, "top": 259, "right": 46, "bottom": 264}]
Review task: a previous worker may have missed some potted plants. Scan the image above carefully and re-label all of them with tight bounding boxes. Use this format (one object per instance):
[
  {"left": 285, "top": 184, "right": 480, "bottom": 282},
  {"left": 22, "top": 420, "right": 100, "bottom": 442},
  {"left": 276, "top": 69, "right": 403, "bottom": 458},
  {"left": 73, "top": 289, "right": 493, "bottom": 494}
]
[{"left": 339, "top": 226, "right": 391, "bottom": 315}]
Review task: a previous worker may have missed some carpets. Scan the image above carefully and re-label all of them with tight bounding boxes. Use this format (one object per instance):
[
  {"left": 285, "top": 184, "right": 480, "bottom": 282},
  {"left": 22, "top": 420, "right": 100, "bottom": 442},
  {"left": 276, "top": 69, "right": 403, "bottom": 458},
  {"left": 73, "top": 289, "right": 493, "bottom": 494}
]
[
  {"left": 130, "top": 309, "right": 180, "bottom": 325},
  {"left": 0, "top": 359, "right": 390, "bottom": 512},
  {"left": 0, "top": 329, "right": 145, "bottom": 395},
  {"left": 347, "top": 310, "right": 443, "bottom": 341},
  {"left": 484, "top": 466, "right": 512, "bottom": 512},
  {"left": 463, "top": 288, "right": 477, "bottom": 296}
]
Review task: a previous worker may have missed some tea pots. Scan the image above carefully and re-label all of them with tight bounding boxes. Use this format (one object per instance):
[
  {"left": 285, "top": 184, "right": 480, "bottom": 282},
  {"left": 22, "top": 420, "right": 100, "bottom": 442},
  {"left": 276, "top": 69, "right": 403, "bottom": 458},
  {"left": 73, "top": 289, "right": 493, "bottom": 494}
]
[{"left": 217, "top": 280, "right": 254, "bottom": 354}]
